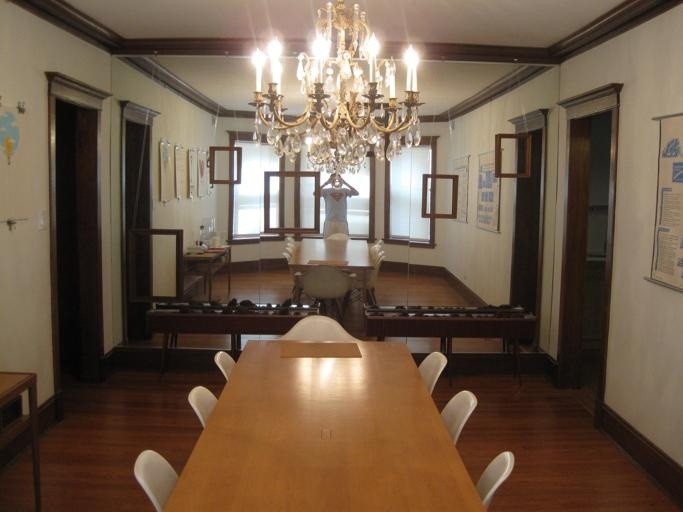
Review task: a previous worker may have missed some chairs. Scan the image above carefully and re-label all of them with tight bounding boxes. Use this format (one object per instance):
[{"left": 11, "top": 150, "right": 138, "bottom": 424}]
[
  {"left": 129, "top": 446, "right": 180, "bottom": 510},
  {"left": 281, "top": 235, "right": 293, "bottom": 268},
  {"left": 372, "top": 240, "right": 389, "bottom": 284},
  {"left": 472, "top": 445, "right": 519, "bottom": 512},
  {"left": 301, "top": 264, "right": 351, "bottom": 315},
  {"left": 281, "top": 314, "right": 364, "bottom": 342},
  {"left": 187, "top": 382, "right": 216, "bottom": 429},
  {"left": 213, "top": 348, "right": 235, "bottom": 381},
  {"left": 411, "top": 352, "right": 448, "bottom": 392},
  {"left": 439, "top": 386, "right": 482, "bottom": 452}
]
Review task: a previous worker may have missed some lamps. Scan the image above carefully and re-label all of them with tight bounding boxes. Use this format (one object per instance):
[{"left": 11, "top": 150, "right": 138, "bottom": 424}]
[{"left": 248, "top": 4, "right": 423, "bottom": 172}]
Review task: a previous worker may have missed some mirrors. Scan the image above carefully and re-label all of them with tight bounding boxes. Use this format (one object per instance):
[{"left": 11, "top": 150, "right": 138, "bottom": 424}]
[{"left": 110, "top": 56, "right": 543, "bottom": 312}]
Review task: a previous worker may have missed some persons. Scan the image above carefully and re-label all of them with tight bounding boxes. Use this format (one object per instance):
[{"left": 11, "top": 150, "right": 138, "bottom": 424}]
[{"left": 313, "top": 172, "right": 360, "bottom": 240}]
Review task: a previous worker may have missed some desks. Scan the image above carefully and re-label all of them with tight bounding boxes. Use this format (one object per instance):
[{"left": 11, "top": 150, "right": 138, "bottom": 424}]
[
  {"left": 292, "top": 237, "right": 372, "bottom": 307},
  {"left": 162, "top": 339, "right": 486, "bottom": 512},
  {"left": 1, "top": 368, "right": 43, "bottom": 511},
  {"left": 183, "top": 243, "right": 234, "bottom": 298}
]
